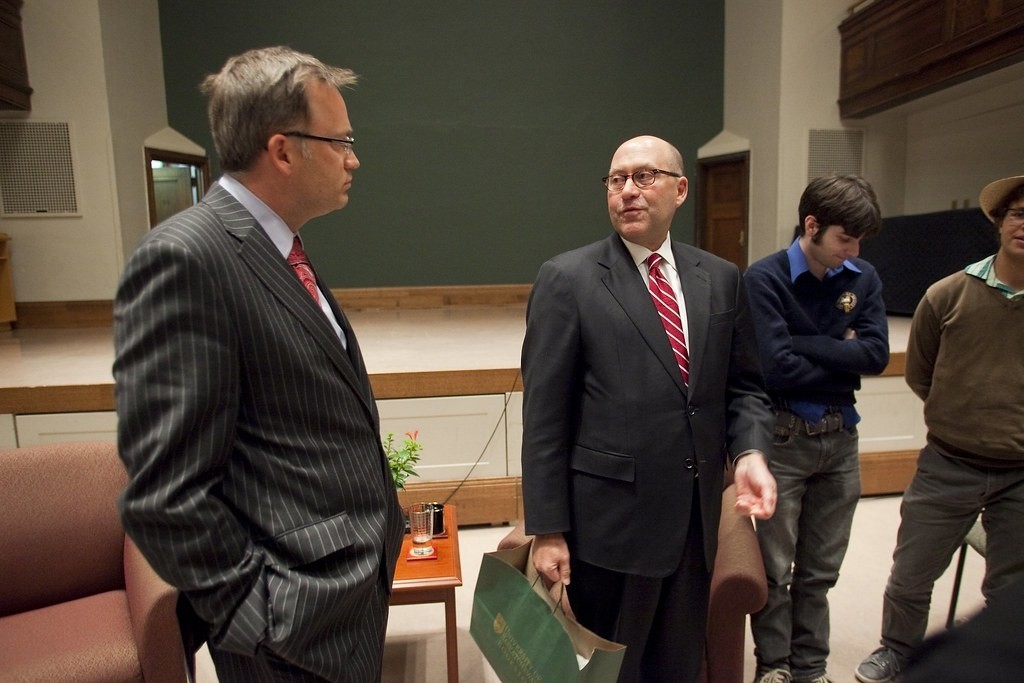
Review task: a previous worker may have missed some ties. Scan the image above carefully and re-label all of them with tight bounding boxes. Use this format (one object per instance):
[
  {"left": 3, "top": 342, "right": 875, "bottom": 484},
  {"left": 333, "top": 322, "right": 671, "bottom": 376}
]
[
  {"left": 286, "top": 236, "right": 318, "bottom": 307},
  {"left": 644, "top": 253, "right": 689, "bottom": 389}
]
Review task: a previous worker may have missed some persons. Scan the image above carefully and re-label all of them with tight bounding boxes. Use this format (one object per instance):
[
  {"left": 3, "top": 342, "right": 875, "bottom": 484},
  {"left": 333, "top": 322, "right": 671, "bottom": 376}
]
[
  {"left": 853, "top": 176, "right": 1024, "bottom": 683},
  {"left": 520, "top": 136, "right": 777, "bottom": 683},
  {"left": 110, "top": 46, "right": 406, "bottom": 682},
  {"left": 742, "top": 171, "right": 891, "bottom": 683}
]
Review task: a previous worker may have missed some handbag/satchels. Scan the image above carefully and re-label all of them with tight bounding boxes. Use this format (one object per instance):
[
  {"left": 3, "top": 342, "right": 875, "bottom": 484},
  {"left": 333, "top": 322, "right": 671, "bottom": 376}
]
[{"left": 469, "top": 536, "right": 626, "bottom": 683}]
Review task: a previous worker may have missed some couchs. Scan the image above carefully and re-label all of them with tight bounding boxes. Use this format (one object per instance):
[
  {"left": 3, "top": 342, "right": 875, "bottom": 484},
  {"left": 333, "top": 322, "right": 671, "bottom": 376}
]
[
  {"left": 496, "top": 455, "right": 770, "bottom": 683},
  {"left": 0, "top": 439, "right": 192, "bottom": 683}
]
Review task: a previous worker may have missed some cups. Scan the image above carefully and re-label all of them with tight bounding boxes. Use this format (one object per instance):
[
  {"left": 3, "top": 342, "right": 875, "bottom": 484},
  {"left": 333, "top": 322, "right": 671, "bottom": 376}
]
[
  {"left": 422, "top": 503, "right": 445, "bottom": 535},
  {"left": 408, "top": 502, "right": 434, "bottom": 557}
]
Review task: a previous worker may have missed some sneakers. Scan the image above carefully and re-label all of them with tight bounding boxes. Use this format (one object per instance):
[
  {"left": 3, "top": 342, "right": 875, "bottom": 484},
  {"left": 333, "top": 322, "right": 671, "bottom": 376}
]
[
  {"left": 855, "top": 646, "right": 911, "bottom": 683},
  {"left": 809, "top": 676, "right": 831, "bottom": 683},
  {"left": 754, "top": 665, "right": 792, "bottom": 683}
]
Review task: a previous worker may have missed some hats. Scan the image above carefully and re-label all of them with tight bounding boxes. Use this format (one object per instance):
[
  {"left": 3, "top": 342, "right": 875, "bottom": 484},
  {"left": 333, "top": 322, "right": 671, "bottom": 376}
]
[{"left": 979, "top": 176, "right": 1024, "bottom": 223}]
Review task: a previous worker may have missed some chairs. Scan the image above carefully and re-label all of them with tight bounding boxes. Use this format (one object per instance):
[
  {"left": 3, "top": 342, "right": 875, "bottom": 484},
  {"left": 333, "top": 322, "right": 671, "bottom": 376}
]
[{"left": 945, "top": 509, "right": 988, "bottom": 629}]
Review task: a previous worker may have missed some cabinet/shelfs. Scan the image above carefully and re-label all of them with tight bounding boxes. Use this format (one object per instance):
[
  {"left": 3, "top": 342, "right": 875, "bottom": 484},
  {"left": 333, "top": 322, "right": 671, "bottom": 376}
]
[{"left": 0, "top": 233, "right": 17, "bottom": 332}]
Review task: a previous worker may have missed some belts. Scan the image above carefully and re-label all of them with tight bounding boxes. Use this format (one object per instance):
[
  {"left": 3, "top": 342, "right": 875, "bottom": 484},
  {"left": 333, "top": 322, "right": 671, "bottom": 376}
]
[{"left": 774, "top": 409, "right": 846, "bottom": 436}]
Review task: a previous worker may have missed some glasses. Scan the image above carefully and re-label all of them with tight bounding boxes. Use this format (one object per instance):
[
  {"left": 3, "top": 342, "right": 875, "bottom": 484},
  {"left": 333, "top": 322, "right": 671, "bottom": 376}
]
[
  {"left": 266, "top": 131, "right": 353, "bottom": 155},
  {"left": 602, "top": 168, "right": 681, "bottom": 192}
]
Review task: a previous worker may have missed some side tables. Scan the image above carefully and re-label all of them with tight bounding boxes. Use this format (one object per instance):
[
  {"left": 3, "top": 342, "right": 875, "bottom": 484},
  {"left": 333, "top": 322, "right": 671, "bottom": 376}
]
[{"left": 386, "top": 503, "right": 463, "bottom": 683}]
